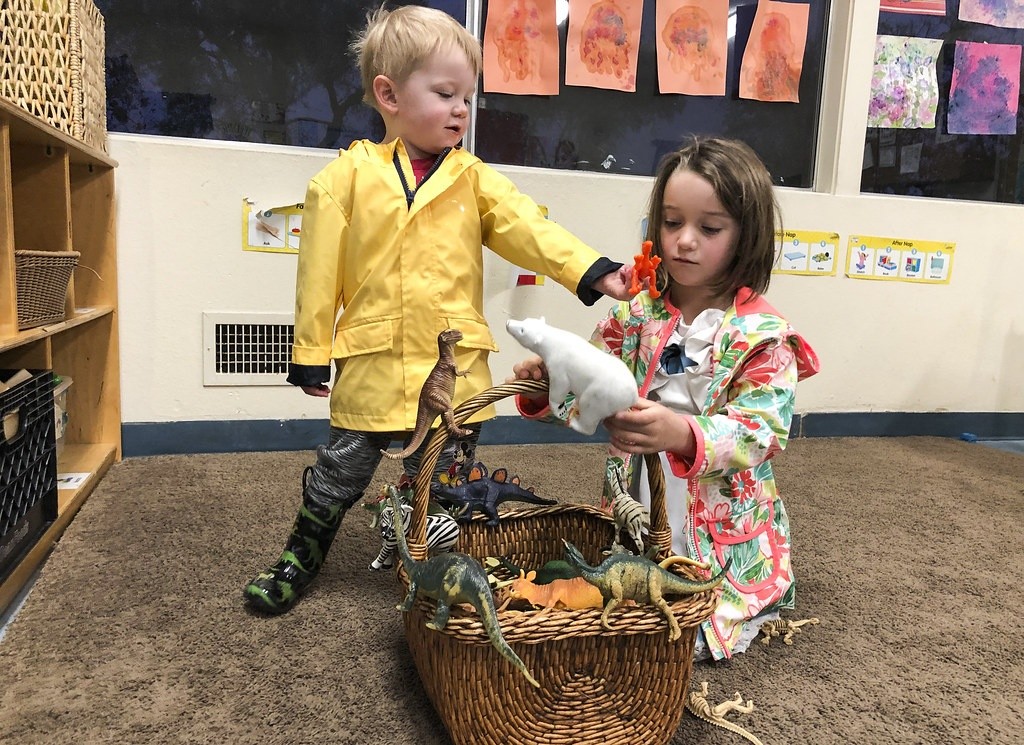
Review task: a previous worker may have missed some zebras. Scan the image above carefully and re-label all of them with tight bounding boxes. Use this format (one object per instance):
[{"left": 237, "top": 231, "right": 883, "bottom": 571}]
[
  {"left": 368, "top": 495, "right": 459, "bottom": 571},
  {"left": 609, "top": 468, "right": 651, "bottom": 556}
]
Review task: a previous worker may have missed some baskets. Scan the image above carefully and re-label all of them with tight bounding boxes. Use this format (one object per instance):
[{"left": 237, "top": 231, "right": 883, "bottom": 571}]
[
  {"left": 392, "top": 379, "right": 720, "bottom": 745},
  {"left": 15, "top": 249, "right": 81, "bottom": 328}
]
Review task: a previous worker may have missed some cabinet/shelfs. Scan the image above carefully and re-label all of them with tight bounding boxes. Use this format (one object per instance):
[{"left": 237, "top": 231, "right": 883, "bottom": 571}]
[{"left": 0, "top": 95, "right": 122, "bottom": 622}]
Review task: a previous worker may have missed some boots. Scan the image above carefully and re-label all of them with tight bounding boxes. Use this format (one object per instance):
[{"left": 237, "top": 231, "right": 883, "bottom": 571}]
[{"left": 244, "top": 466, "right": 365, "bottom": 613}]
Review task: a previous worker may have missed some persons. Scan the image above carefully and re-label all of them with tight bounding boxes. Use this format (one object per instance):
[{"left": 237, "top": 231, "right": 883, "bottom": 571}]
[
  {"left": 505, "top": 134, "right": 820, "bottom": 664},
  {"left": 242, "top": 4, "right": 651, "bottom": 619}
]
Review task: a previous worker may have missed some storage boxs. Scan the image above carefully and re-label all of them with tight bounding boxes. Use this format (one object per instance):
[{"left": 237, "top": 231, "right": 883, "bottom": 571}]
[{"left": 0, "top": 369, "right": 58, "bottom": 586}]
